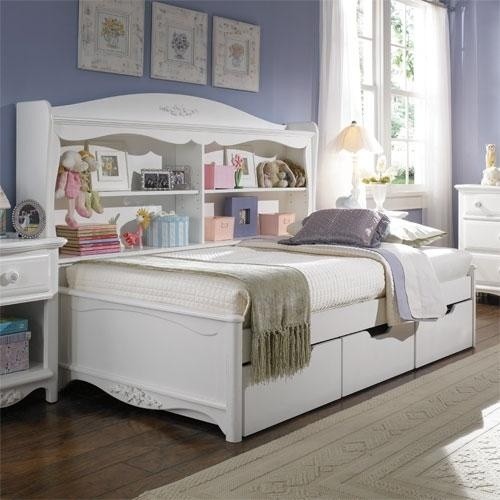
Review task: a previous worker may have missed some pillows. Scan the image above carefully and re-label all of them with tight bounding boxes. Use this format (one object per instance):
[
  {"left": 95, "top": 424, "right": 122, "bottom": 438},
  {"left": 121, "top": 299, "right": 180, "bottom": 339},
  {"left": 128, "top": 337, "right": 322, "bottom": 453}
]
[
  {"left": 380, "top": 217, "right": 448, "bottom": 248},
  {"left": 277, "top": 209, "right": 390, "bottom": 249}
]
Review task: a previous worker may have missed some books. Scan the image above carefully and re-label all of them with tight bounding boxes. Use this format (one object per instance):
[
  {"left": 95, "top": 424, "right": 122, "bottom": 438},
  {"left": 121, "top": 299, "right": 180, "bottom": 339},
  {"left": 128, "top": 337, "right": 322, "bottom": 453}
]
[{"left": 56, "top": 223, "right": 121, "bottom": 256}]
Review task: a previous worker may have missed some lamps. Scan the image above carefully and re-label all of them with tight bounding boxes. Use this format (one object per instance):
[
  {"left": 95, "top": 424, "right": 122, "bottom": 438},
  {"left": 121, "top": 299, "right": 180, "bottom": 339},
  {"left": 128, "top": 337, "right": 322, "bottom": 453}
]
[{"left": 324, "top": 121, "right": 383, "bottom": 210}]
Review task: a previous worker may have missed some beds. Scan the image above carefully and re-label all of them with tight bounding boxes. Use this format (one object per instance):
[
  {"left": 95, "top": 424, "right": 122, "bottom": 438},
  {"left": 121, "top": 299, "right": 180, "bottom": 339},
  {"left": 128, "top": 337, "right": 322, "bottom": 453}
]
[{"left": 57, "top": 204, "right": 478, "bottom": 443}]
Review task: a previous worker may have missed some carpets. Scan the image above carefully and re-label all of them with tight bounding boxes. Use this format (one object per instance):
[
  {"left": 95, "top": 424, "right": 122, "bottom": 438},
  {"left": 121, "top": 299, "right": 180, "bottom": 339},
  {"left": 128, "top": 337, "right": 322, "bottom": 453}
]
[{"left": 132, "top": 343, "right": 500, "bottom": 500}]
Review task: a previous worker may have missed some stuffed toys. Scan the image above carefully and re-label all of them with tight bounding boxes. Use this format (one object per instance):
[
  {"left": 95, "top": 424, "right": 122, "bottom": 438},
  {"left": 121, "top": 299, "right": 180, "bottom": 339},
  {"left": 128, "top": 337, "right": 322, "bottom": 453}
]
[
  {"left": 256, "top": 159, "right": 296, "bottom": 188},
  {"left": 55, "top": 150, "right": 91, "bottom": 228},
  {"left": 279, "top": 158, "right": 306, "bottom": 187},
  {"left": 77, "top": 151, "right": 104, "bottom": 218}
]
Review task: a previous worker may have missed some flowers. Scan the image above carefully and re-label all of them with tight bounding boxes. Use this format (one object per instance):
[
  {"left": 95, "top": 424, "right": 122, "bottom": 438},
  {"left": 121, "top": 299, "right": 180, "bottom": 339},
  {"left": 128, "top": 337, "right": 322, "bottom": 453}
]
[{"left": 232, "top": 154, "right": 245, "bottom": 188}]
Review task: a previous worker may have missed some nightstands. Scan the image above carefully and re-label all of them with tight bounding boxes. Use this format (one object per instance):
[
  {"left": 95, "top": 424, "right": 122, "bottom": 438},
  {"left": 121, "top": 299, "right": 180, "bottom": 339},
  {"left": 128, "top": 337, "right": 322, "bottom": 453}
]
[{"left": 0, "top": 231, "right": 68, "bottom": 409}]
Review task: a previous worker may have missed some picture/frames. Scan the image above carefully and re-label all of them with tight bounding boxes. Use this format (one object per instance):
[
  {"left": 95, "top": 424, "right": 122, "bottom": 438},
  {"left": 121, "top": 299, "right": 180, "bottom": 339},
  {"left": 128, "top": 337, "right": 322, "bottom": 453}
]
[
  {"left": 11, "top": 199, "right": 46, "bottom": 239},
  {"left": 141, "top": 169, "right": 175, "bottom": 191},
  {"left": 87, "top": 141, "right": 131, "bottom": 192},
  {"left": 76, "top": 0, "right": 145, "bottom": 78},
  {"left": 212, "top": 15, "right": 262, "bottom": 93},
  {"left": 224, "top": 145, "right": 258, "bottom": 188},
  {"left": 165, "top": 165, "right": 190, "bottom": 191},
  {"left": 150, "top": 2, "right": 208, "bottom": 86}
]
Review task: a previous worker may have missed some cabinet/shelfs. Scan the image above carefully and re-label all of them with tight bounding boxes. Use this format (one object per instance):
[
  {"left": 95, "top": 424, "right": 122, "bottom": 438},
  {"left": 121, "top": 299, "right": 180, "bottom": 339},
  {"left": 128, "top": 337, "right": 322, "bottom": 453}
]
[
  {"left": 15, "top": 92, "right": 320, "bottom": 265},
  {"left": 453, "top": 183, "right": 500, "bottom": 296}
]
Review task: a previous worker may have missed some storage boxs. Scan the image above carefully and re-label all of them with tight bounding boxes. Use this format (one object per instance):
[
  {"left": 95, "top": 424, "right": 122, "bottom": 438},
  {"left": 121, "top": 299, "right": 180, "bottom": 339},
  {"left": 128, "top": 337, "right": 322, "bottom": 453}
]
[
  {"left": 0, "top": 330, "right": 32, "bottom": 375},
  {"left": 205, "top": 216, "right": 235, "bottom": 241},
  {"left": 204, "top": 163, "right": 236, "bottom": 190},
  {"left": 259, "top": 212, "right": 295, "bottom": 236}
]
